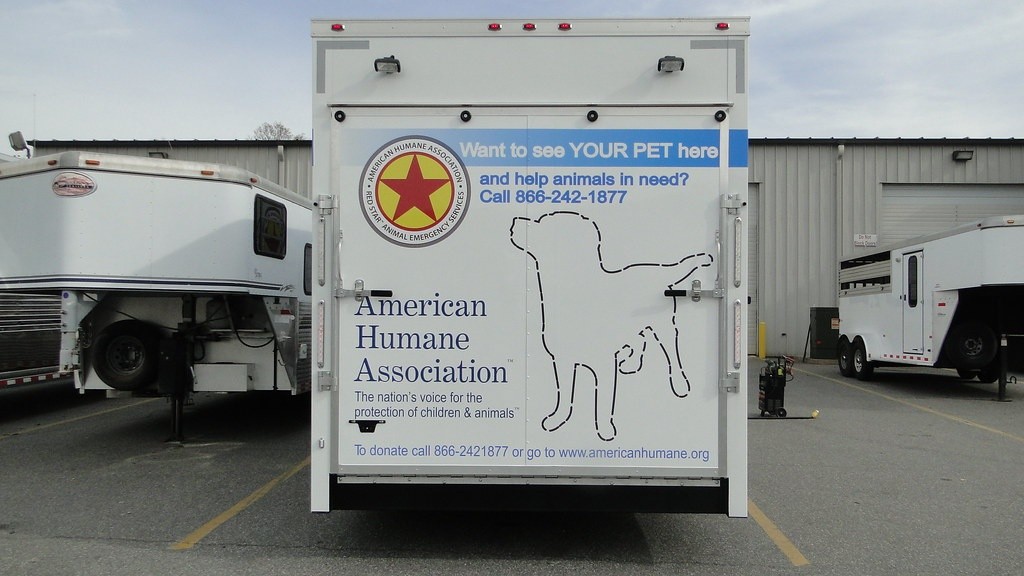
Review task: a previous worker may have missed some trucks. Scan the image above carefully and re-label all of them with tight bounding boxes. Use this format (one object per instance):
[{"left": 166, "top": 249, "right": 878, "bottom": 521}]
[{"left": 306, "top": 16, "right": 752, "bottom": 515}]
